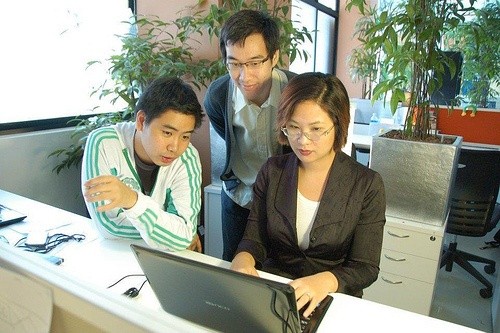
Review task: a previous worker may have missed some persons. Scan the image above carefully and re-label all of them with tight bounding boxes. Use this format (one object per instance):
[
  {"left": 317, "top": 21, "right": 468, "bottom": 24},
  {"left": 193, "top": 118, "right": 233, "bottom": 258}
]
[
  {"left": 231, "top": 70, "right": 386, "bottom": 316},
  {"left": 81, "top": 76, "right": 205, "bottom": 252},
  {"left": 204, "top": 9, "right": 299, "bottom": 263}
]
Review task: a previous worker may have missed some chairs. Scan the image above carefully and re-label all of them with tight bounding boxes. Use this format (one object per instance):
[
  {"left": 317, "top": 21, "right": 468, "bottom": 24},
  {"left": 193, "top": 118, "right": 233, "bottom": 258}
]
[{"left": 438, "top": 149, "right": 500, "bottom": 299}]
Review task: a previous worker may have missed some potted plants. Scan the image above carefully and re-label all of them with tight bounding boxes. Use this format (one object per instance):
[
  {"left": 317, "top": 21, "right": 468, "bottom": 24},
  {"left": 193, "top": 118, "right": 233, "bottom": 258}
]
[{"left": 344, "top": 0, "right": 488, "bottom": 225}]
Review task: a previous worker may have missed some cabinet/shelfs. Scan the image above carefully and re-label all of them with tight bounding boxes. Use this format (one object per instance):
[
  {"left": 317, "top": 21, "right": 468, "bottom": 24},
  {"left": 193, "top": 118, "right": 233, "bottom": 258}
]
[{"left": 362, "top": 210, "right": 449, "bottom": 315}]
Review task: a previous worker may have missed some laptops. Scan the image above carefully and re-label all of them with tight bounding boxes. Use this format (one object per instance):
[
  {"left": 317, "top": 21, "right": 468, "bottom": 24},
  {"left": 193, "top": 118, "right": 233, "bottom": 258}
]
[
  {"left": 130, "top": 243, "right": 334, "bottom": 333},
  {"left": 0, "top": 204, "right": 27, "bottom": 227}
]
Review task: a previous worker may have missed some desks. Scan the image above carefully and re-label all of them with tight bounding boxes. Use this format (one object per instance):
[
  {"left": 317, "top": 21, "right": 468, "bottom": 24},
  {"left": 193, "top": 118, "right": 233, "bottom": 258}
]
[
  {"left": 0, "top": 189, "right": 490, "bottom": 332},
  {"left": 346, "top": 106, "right": 500, "bottom": 158}
]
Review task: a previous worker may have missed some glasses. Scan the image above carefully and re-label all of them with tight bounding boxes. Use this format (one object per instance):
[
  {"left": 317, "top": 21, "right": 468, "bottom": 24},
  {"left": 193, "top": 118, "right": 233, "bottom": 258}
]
[
  {"left": 225, "top": 55, "right": 270, "bottom": 70},
  {"left": 281, "top": 121, "right": 334, "bottom": 141}
]
